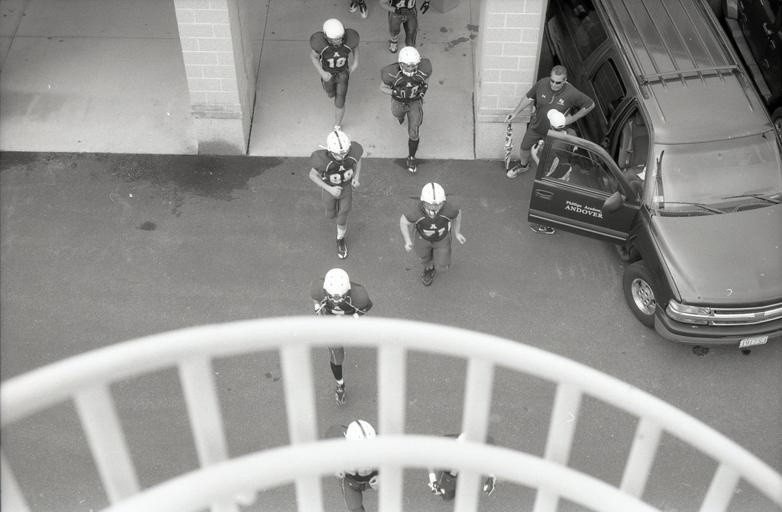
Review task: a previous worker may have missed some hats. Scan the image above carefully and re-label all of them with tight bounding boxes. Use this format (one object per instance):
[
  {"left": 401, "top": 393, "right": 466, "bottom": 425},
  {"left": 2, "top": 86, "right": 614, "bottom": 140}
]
[{"left": 547, "top": 109, "right": 566, "bottom": 130}]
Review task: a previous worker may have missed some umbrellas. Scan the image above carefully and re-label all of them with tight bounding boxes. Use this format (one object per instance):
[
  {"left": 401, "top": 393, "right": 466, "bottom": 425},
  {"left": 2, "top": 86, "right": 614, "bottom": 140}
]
[{"left": 504, "top": 115, "right": 513, "bottom": 173}]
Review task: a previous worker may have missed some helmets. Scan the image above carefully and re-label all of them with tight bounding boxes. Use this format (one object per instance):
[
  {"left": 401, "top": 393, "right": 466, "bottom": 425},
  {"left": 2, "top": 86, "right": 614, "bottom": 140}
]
[
  {"left": 323, "top": 18, "right": 345, "bottom": 38},
  {"left": 398, "top": 46, "right": 420, "bottom": 76},
  {"left": 345, "top": 420, "right": 375, "bottom": 437},
  {"left": 327, "top": 131, "right": 350, "bottom": 161},
  {"left": 323, "top": 268, "right": 351, "bottom": 297},
  {"left": 419, "top": 182, "right": 446, "bottom": 205}
]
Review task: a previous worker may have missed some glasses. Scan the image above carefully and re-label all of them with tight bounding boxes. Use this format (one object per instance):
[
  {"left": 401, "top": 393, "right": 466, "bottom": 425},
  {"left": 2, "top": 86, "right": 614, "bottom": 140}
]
[{"left": 549, "top": 78, "right": 562, "bottom": 84}]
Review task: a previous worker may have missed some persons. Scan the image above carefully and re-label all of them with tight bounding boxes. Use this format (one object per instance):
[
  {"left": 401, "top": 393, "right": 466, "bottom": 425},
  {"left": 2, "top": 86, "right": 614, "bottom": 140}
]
[
  {"left": 379, "top": 0, "right": 430, "bottom": 54},
  {"left": 429, "top": 434, "right": 497, "bottom": 502},
  {"left": 380, "top": 46, "right": 432, "bottom": 176},
  {"left": 349, "top": 0, "right": 368, "bottom": 18},
  {"left": 311, "top": 268, "right": 373, "bottom": 406},
  {"left": 504, "top": 65, "right": 596, "bottom": 179},
  {"left": 310, "top": 17, "right": 360, "bottom": 131},
  {"left": 528, "top": 109, "right": 578, "bottom": 235},
  {"left": 308, "top": 130, "right": 364, "bottom": 259},
  {"left": 399, "top": 182, "right": 466, "bottom": 287},
  {"left": 326, "top": 419, "right": 379, "bottom": 512}
]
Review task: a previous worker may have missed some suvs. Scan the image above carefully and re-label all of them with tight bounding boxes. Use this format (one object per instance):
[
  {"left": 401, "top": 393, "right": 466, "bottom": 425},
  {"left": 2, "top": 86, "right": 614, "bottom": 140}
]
[{"left": 528, "top": 2, "right": 782, "bottom": 347}]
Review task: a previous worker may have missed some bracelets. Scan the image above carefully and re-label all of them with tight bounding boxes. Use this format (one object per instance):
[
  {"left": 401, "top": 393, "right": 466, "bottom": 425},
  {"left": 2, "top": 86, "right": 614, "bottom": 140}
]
[
  {"left": 315, "top": 304, "right": 321, "bottom": 311},
  {"left": 429, "top": 473, "right": 436, "bottom": 483},
  {"left": 353, "top": 313, "right": 358, "bottom": 316}
]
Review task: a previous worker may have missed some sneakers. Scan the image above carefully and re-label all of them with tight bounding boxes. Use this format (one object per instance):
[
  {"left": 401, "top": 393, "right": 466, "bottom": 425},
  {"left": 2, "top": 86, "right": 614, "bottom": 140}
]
[
  {"left": 349, "top": 0, "right": 368, "bottom": 18},
  {"left": 406, "top": 156, "right": 416, "bottom": 173},
  {"left": 336, "top": 384, "right": 346, "bottom": 406},
  {"left": 529, "top": 223, "right": 555, "bottom": 235},
  {"left": 388, "top": 40, "right": 397, "bottom": 52},
  {"left": 336, "top": 234, "right": 348, "bottom": 260},
  {"left": 423, "top": 265, "right": 435, "bottom": 287},
  {"left": 507, "top": 161, "right": 530, "bottom": 178}
]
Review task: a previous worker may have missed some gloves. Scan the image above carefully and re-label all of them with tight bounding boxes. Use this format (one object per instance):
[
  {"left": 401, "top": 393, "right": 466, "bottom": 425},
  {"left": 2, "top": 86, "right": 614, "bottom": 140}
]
[
  {"left": 393, "top": 83, "right": 428, "bottom": 100},
  {"left": 420, "top": 1, "right": 429, "bottom": 14},
  {"left": 483, "top": 478, "right": 494, "bottom": 495},
  {"left": 428, "top": 481, "right": 441, "bottom": 494},
  {"left": 395, "top": 6, "right": 408, "bottom": 15}
]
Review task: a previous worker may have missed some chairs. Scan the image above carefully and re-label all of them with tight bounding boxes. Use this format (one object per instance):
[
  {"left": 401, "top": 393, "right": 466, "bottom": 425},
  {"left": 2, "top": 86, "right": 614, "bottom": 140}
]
[{"left": 618, "top": 111, "right": 650, "bottom": 176}]
